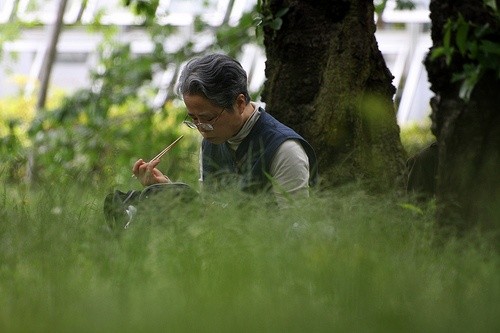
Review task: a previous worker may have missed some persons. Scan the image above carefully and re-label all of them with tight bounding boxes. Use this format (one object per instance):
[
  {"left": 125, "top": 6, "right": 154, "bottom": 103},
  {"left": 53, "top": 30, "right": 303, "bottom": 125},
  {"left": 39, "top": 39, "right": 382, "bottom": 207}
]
[{"left": 103, "top": 54, "right": 319, "bottom": 257}]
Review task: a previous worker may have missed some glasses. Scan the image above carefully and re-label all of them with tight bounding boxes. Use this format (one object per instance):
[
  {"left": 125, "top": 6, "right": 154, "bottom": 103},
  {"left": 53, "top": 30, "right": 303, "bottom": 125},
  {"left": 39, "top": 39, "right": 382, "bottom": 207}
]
[{"left": 181, "top": 106, "right": 226, "bottom": 132}]
